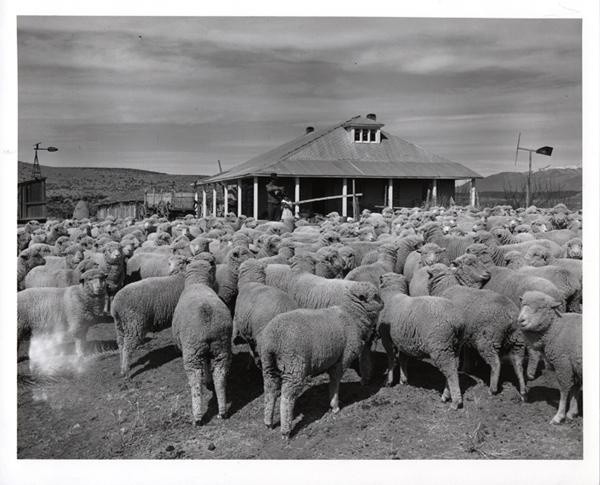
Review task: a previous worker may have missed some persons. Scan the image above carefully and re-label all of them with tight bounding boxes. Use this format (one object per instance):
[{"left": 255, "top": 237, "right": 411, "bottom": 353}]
[{"left": 280, "top": 191, "right": 293, "bottom": 220}]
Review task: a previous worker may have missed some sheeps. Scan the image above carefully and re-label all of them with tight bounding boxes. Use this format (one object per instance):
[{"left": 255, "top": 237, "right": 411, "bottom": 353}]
[{"left": 17, "top": 205, "right": 582, "bottom": 440}]
